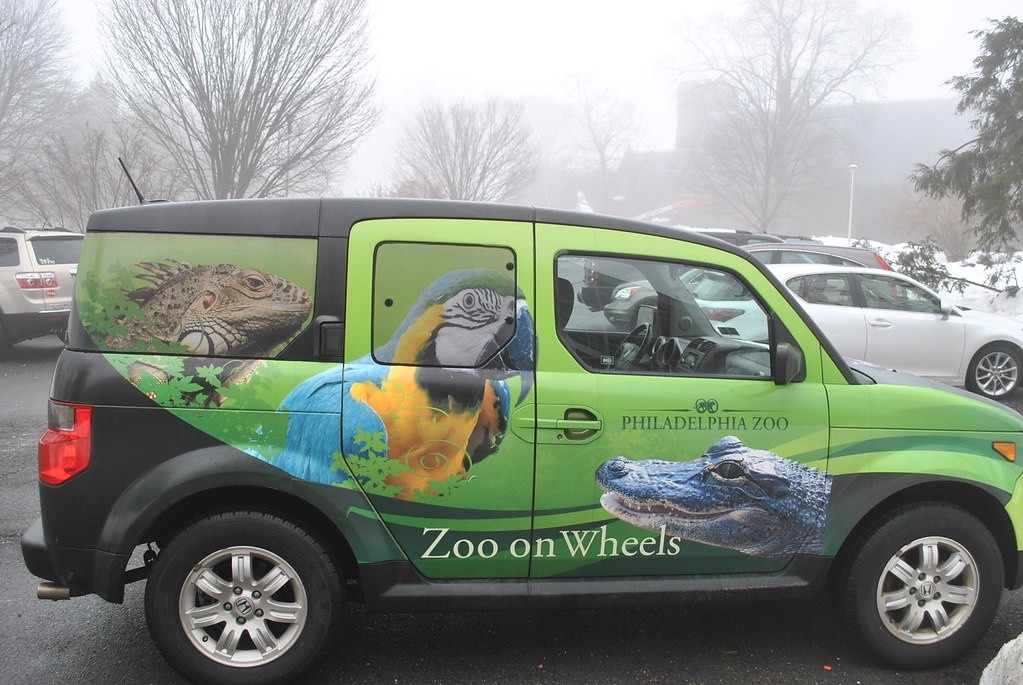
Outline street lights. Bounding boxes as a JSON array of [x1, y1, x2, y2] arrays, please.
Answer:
[[847, 162, 859, 247]]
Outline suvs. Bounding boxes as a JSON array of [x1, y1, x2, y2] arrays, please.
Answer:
[[686, 261, 1023, 401], [575, 227, 828, 312], [0, 224, 85, 365], [11, 194, 1023, 685], [601, 237, 902, 333]]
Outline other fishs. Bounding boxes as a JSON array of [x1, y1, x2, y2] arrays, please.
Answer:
[[595, 436, 833, 558]]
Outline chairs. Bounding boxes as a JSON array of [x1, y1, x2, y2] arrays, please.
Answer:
[[840, 284, 854, 305], [811, 277, 828, 303], [559, 278, 576, 352]]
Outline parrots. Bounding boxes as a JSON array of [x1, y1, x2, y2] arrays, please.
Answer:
[[240, 269, 539, 500]]
